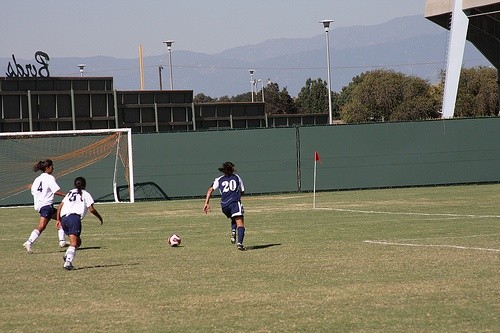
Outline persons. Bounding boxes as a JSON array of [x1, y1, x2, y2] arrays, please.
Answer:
[[23, 159, 71, 253], [55, 177, 103, 270], [203, 162, 247, 251]]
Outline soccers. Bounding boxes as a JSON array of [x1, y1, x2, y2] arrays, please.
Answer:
[[168, 234, 182, 247]]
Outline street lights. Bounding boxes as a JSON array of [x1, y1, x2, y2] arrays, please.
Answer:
[[318, 20, 335, 124], [258, 79, 264, 101], [248, 70, 254, 102], [77, 64, 86, 77], [162, 41, 175, 90]]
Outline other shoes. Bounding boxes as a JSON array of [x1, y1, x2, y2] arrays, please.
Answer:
[[237, 242, 246, 251], [63, 255, 66, 261], [23, 239, 33, 254], [59, 241, 71, 248], [230, 231, 236, 244], [64, 261, 74, 270]]
